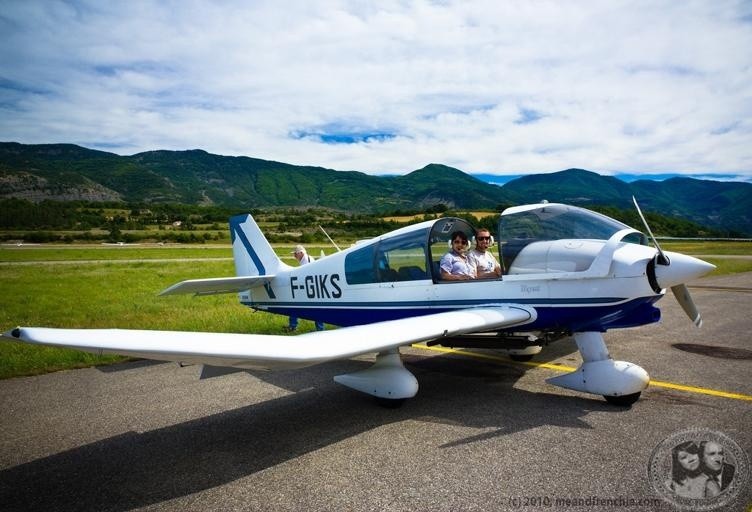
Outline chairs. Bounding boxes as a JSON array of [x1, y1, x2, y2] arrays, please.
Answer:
[[428, 260, 441, 277], [399, 265, 423, 280]]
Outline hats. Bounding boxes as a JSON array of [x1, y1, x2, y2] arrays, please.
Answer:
[[289, 244, 304, 254]]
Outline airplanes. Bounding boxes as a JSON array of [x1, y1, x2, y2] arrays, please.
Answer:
[[0, 195, 716, 410]]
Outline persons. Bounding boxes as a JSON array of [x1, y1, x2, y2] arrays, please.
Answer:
[[670, 440, 721, 498], [470, 228, 504, 280], [697, 439, 733, 492], [286, 244, 325, 332], [438, 230, 474, 280]]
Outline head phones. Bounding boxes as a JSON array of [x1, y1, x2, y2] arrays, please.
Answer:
[[472, 236, 495, 245], [448, 239, 471, 249]]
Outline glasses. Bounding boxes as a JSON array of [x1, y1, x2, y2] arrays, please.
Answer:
[[476, 236, 490, 241], [455, 240, 466, 245]]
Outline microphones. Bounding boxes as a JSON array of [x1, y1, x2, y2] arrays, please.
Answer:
[[485, 245, 494, 249], [458, 248, 467, 251]]
